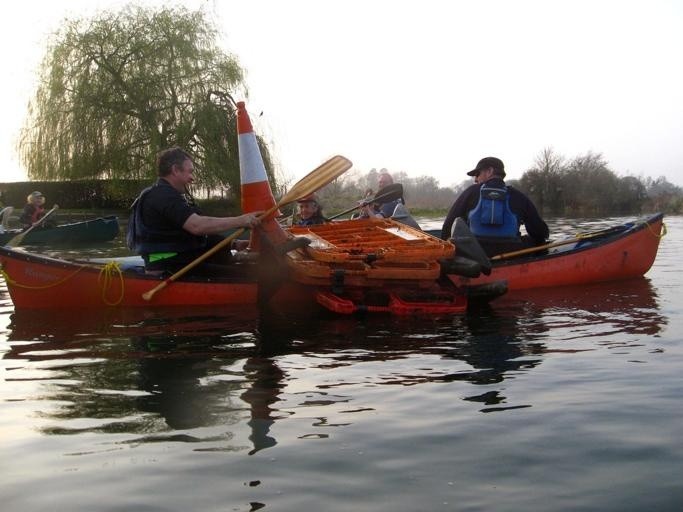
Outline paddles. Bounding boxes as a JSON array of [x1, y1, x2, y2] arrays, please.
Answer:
[[488, 222, 634, 262], [5, 206, 57, 246], [142, 156, 353, 301], [326, 183, 403, 220]]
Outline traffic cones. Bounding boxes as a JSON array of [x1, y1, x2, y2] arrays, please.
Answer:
[[234, 100, 311, 265]]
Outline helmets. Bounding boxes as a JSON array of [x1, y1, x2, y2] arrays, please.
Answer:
[[296, 190, 318, 203]]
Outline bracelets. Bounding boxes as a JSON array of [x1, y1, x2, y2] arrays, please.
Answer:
[[232, 238, 240, 248]]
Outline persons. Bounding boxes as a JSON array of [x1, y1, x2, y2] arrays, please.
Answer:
[[18, 192, 57, 230], [438, 157, 550, 262], [124, 147, 267, 278], [293, 191, 330, 225], [349, 172, 402, 222]]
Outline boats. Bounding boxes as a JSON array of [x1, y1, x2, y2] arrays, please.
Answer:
[[0, 210, 668, 310], [0, 214, 122, 248]]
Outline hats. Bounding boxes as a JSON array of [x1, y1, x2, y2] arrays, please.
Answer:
[[466, 156, 504, 176], [29, 191, 44, 199]]
